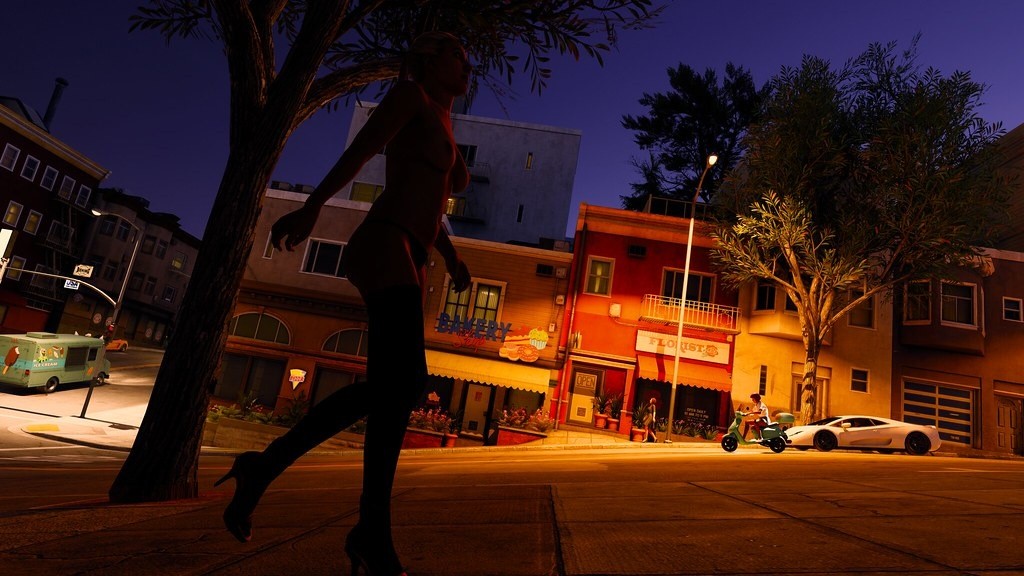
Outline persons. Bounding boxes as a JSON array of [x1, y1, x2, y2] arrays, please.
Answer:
[[743, 394, 769, 440], [212, 31, 472, 576], [643, 397, 658, 443]]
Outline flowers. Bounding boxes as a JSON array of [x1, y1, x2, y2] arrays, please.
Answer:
[[657, 417, 718, 439], [407, 404, 460, 435], [493, 403, 556, 433]]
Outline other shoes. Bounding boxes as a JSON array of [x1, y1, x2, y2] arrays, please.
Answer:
[[654, 438, 658, 443], [643, 438, 648, 443]]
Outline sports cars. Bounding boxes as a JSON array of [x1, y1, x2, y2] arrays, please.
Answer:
[[785, 415, 942, 455]]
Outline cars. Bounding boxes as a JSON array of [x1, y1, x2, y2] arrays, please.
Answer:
[[92, 335, 128, 353]]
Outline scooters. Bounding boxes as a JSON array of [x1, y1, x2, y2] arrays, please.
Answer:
[[720, 404, 796, 453]]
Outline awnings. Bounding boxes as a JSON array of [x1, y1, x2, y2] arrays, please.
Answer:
[[634, 355, 732, 392], [425, 348, 550, 395]]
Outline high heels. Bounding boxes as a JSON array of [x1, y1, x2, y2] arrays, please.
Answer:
[[345, 524, 411, 576], [214, 451, 265, 543]]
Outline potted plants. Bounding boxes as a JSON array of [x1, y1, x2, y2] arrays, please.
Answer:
[[202, 389, 369, 452], [590, 391, 645, 443]]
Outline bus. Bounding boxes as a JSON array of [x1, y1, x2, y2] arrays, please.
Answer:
[[0, 332, 112, 393]]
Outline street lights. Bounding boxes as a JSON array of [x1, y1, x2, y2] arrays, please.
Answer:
[[79, 209, 144, 420], [661, 153, 719, 442]]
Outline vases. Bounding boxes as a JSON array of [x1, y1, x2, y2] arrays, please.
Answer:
[[496, 425, 548, 446], [401, 426, 459, 450]]
